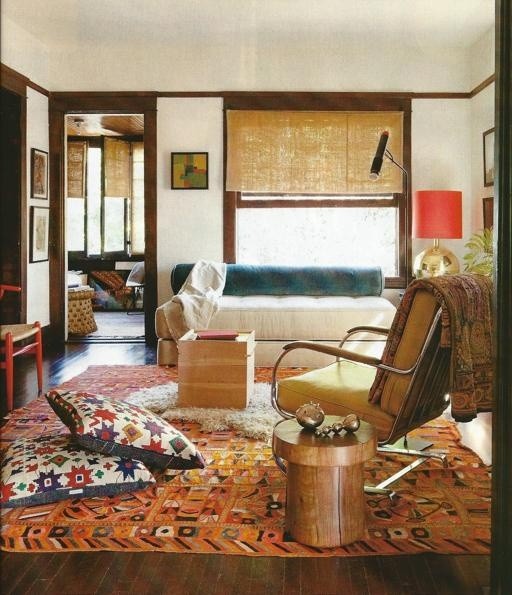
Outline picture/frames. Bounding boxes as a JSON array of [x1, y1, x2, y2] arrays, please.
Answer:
[[172, 152, 208, 189], [483, 198, 494, 256], [30, 207, 49, 263], [483, 127, 495, 187], [31, 149, 48, 200]]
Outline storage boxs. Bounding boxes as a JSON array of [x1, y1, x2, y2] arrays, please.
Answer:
[[176, 329, 257, 409]]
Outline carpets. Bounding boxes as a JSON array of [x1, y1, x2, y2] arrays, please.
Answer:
[[123, 382, 288, 443], [0, 364, 492, 559]]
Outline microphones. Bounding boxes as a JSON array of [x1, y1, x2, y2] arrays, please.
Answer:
[[370, 132, 390, 181]]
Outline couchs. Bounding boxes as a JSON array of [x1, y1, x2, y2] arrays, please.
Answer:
[[154, 264, 397, 374]]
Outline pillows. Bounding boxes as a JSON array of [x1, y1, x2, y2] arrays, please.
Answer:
[[0, 437, 157, 508], [45, 388, 207, 470]]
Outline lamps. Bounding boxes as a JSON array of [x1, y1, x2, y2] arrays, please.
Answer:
[[412, 190, 462, 280], [369, 131, 409, 299]]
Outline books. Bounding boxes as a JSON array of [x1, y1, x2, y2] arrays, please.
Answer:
[[197, 330, 239, 339]]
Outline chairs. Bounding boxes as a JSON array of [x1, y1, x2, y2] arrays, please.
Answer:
[[0, 285, 42, 410], [271, 271, 492, 506]]
